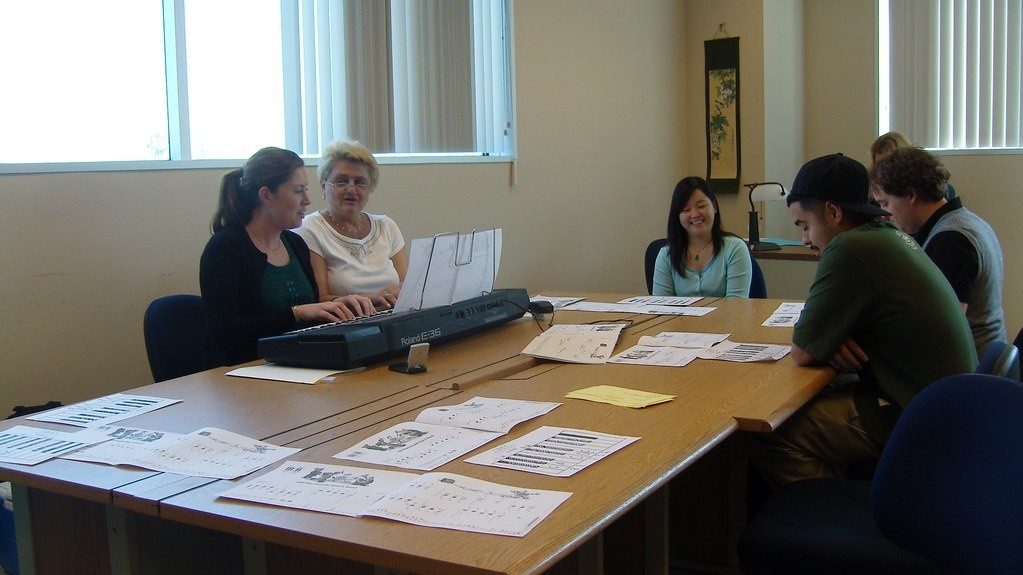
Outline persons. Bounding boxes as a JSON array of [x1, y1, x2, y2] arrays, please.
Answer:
[[869, 146, 1008, 360], [871, 131, 955, 201], [291, 141, 408, 309], [748, 153, 978, 482], [199, 147, 377, 363], [652, 177, 752, 299]]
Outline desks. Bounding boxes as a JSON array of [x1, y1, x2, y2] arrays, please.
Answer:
[[752, 245, 819, 261], [0, 290, 834, 575]]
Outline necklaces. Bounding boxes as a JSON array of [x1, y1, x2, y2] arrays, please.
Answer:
[[327, 209, 363, 232], [692, 239, 712, 260]]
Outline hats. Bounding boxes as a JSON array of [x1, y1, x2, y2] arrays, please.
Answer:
[[791, 153, 893, 216]]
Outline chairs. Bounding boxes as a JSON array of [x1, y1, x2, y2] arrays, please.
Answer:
[[645, 238, 767, 298], [144, 294, 202, 382], [747, 343, 1019, 550], [872, 377, 1023, 575]]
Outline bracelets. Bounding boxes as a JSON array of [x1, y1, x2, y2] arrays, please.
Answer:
[[331, 297, 339, 302]]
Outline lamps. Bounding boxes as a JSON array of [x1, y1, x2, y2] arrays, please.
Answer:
[[743, 183, 792, 249]]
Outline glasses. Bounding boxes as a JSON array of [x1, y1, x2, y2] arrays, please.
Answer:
[[326, 181, 372, 194]]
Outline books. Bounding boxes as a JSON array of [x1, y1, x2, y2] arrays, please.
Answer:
[[520, 324, 626, 365]]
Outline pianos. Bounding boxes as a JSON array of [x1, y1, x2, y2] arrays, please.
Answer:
[[256, 288, 531, 370]]
